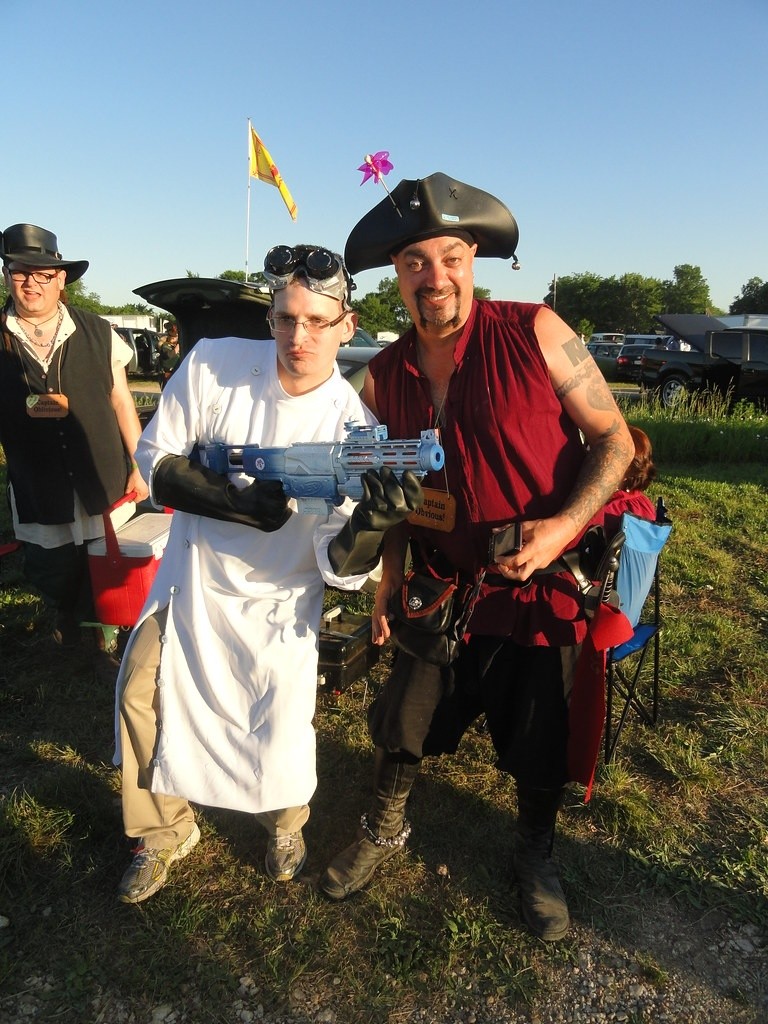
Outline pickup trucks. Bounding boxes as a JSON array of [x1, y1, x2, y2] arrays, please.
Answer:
[[639, 314, 767, 410]]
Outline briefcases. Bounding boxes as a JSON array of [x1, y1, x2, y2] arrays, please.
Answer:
[[87, 492, 174, 627], [317, 605, 380, 695]]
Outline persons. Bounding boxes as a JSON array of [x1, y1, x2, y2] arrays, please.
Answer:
[[638, 337, 691, 394], [578, 333, 587, 348], [610, 335, 617, 343], [135, 335, 148, 380], [156, 322, 180, 389], [0, 224, 149, 669], [113, 246, 425, 902], [585, 425, 659, 652], [109, 323, 129, 375], [318, 171, 635, 941]]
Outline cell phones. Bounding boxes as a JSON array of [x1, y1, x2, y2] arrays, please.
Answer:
[[487, 522, 523, 564]]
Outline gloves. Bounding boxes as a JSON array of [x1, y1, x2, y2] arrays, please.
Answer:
[[153, 454, 294, 533], [328, 467, 425, 577]]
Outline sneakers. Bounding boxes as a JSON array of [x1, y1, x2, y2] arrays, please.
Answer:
[[511, 847, 570, 941], [117, 822, 201, 903], [265, 829, 307, 882], [319, 836, 405, 900]]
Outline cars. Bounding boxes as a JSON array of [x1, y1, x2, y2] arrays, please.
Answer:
[[113, 327, 164, 375], [615, 344, 658, 382], [131, 278, 387, 377], [585, 341, 624, 381]]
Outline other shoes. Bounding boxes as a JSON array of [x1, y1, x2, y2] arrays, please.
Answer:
[[117, 629, 134, 659], [57, 615, 103, 674]]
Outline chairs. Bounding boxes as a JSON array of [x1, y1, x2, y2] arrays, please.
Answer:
[[603, 495, 673, 766]]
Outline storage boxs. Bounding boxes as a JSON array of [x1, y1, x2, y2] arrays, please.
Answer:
[[88, 513, 173, 627]]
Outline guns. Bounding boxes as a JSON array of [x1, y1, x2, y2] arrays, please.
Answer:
[[188, 412, 447, 519]]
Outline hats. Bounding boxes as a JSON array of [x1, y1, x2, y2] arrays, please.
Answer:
[[0, 224, 89, 285], [344, 171, 522, 290]]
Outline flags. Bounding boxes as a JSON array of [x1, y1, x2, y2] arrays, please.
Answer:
[[248, 124, 297, 221]]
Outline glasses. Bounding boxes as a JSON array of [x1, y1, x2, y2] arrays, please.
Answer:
[[8, 269, 62, 284], [266, 304, 348, 334], [263, 245, 341, 288]]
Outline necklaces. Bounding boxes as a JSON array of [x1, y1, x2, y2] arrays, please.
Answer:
[[15, 301, 63, 347]]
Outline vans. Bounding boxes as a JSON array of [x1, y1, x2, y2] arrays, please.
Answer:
[[589, 333, 625, 344]]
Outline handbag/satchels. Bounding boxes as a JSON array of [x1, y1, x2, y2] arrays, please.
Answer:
[[386, 571, 458, 639]]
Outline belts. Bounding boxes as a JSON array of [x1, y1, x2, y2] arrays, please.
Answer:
[[429, 547, 583, 584]]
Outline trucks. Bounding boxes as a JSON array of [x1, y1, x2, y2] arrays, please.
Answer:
[[377, 332, 399, 347], [98, 314, 169, 333]]
